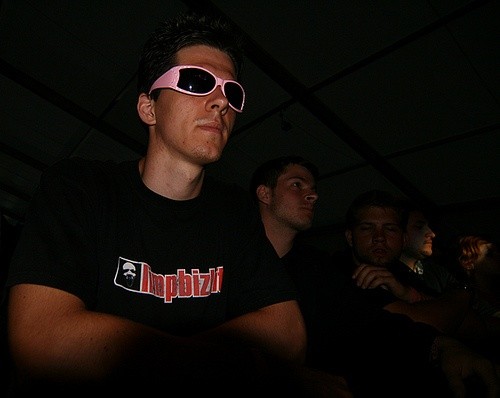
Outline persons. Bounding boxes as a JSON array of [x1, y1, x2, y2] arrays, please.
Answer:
[[383, 199, 471, 305], [450, 230, 500, 332], [218, 154, 499, 398], [2, 16, 308, 397], [337, 187, 473, 333]]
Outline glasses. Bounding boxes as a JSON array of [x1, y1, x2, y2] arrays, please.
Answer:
[[149, 64, 246, 113]]
